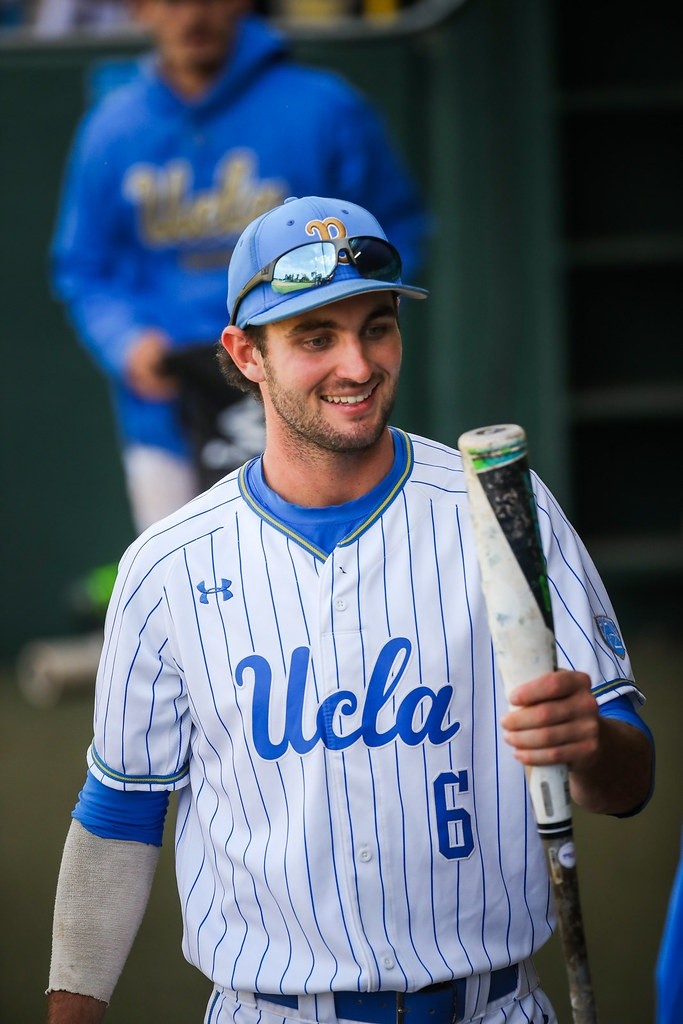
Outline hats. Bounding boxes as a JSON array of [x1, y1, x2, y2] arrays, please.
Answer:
[[227, 194, 427, 329]]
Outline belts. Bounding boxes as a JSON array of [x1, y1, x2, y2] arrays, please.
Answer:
[[252, 961, 519, 1024]]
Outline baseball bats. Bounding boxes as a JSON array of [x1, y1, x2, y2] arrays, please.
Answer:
[[457, 424, 598, 1024]]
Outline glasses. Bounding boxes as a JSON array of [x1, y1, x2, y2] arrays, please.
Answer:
[[230, 236, 404, 328]]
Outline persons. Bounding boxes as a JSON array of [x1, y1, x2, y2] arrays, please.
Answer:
[[49, 0, 427, 540], [46, 195, 656, 1024]]
